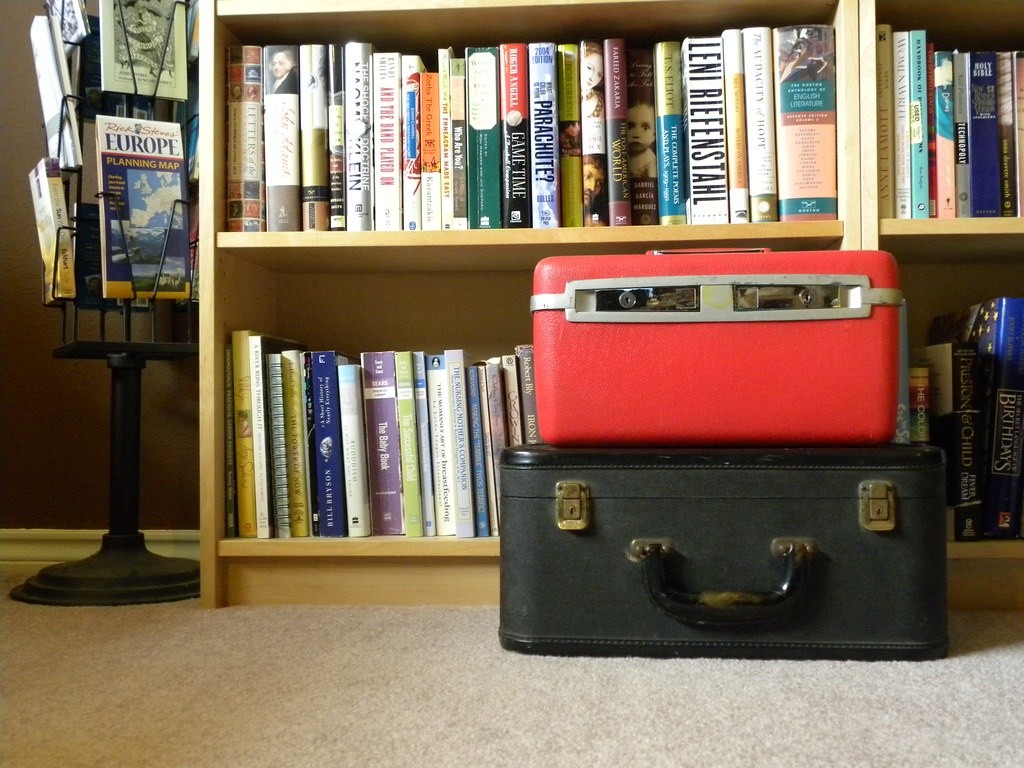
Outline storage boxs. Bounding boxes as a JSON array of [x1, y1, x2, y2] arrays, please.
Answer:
[[530, 247, 902, 447]]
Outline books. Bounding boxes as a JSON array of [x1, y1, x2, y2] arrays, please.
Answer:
[[28, 0, 91, 308], [97, 0, 189, 103], [94, 114, 192, 299], [227, 24, 839, 234], [222, 326, 542, 537], [876, 22, 1024, 219], [909, 342, 1023, 542]]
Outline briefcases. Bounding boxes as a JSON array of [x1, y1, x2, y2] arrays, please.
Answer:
[[499, 446, 951, 662], [530, 242, 906, 446]]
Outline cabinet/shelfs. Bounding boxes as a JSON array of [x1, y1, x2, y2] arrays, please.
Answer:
[[9, 0, 199, 606], [199, 0, 1024, 609]]
